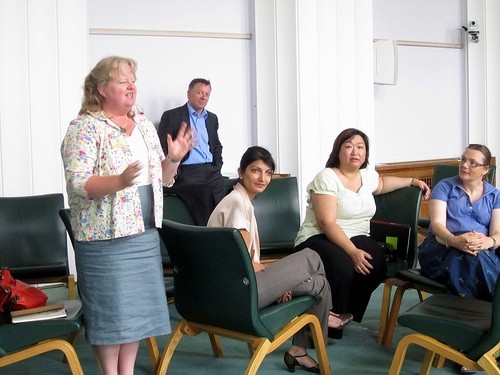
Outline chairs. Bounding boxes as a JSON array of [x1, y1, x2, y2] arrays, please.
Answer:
[[0, 164, 500, 375]]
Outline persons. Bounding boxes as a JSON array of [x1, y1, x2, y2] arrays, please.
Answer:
[[293, 129, 432, 340], [60, 56, 201, 375], [158, 79, 226, 226], [417, 144, 500, 375], [205, 146, 354, 372]]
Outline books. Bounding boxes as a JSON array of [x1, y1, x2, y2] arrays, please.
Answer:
[[10, 304, 67, 323]]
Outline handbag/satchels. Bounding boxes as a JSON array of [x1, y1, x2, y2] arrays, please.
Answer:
[[0, 267, 48, 313]]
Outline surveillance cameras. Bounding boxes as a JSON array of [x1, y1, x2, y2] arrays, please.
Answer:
[[468, 20, 479, 31]]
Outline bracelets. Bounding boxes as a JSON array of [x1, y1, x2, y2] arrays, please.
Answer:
[[168, 157, 180, 163], [491, 237, 496, 248], [410, 178, 414, 187]]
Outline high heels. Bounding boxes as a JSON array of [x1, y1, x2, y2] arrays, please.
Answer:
[[284, 351, 331, 372], [328, 311, 354, 330]]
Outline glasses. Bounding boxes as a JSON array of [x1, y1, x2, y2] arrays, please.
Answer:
[[458, 157, 487, 167]]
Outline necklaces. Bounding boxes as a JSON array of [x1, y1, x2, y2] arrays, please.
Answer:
[[104, 111, 127, 133]]
